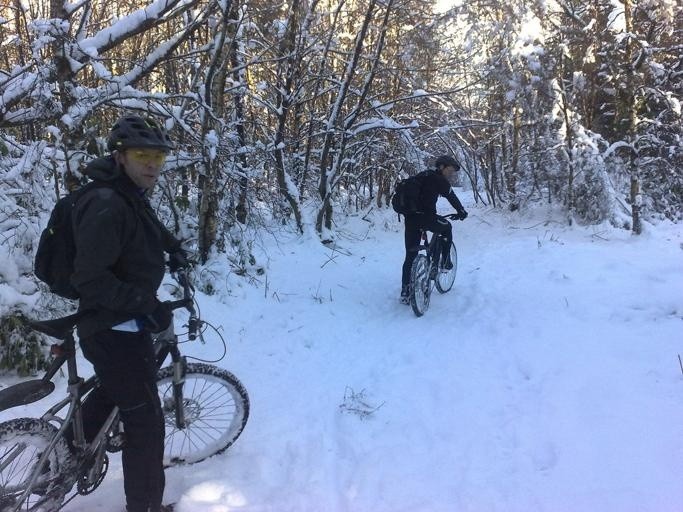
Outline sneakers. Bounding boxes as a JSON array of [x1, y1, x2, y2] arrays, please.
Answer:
[[22, 462, 50, 497], [399, 292, 411, 305], [441, 262, 452, 272]]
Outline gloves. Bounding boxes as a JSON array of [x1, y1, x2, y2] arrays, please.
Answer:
[[168, 247, 188, 270], [135, 299, 173, 336], [456, 207, 468, 220]]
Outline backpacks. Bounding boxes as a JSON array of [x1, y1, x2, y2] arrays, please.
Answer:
[[391, 169, 437, 221], [34, 182, 118, 302]]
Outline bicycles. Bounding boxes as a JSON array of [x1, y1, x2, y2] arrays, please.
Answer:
[[0, 259, 250, 512], [407, 214, 458, 317]]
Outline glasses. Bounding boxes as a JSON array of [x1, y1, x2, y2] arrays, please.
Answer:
[[123, 151, 167, 166]]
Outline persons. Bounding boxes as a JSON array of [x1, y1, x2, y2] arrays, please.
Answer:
[[399, 155, 468, 306], [67, 115, 195, 512]]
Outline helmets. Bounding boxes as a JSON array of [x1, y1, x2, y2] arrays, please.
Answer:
[[107, 115, 177, 151], [434, 156, 460, 172]]
